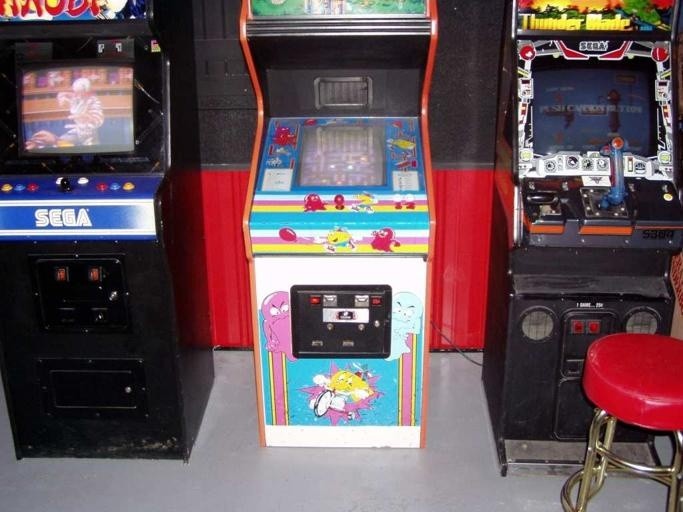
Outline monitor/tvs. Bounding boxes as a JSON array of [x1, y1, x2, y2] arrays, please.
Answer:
[[530, 53, 659, 159], [289, 117, 390, 191], [16, 61, 139, 157]]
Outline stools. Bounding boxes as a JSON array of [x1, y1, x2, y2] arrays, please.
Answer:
[[560, 332, 683, 508]]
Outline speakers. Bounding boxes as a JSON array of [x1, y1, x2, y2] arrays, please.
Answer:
[[501, 274, 675, 442]]
